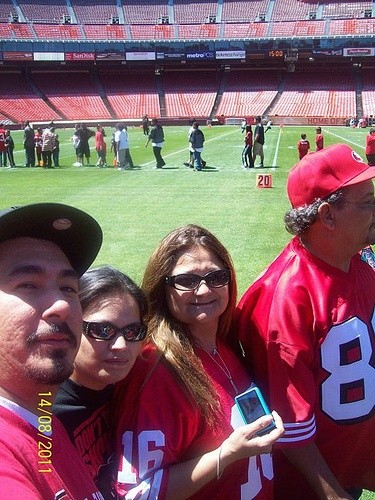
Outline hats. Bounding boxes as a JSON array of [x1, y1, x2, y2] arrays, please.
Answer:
[[0, 202, 104, 278], [287, 144, 375, 209]]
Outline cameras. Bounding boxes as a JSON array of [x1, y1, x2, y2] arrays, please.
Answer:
[[235, 387, 275, 438]]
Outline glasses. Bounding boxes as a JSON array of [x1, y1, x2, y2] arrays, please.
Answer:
[[164, 270, 231, 291], [81, 320, 148, 342], [340, 200, 375, 209]]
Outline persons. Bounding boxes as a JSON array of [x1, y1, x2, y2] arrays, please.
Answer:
[[0, 203, 105, 500], [71, 123, 96, 166], [145, 118, 165, 169], [350, 116, 367, 129], [53, 266, 148, 500], [109, 225, 285, 500], [4, 130, 15, 168], [236, 144, 375, 500], [264, 119, 273, 133], [183, 119, 206, 172], [365, 128, 375, 167], [23, 120, 60, 168], [315, 126, 323, 152], [110, 123, 133, 168], [142, 115, 150, 136], [298, 133, 310, 160], [242, 116, 265, 168], [0, 123, 8, 167], [95, 124, 107, 168]]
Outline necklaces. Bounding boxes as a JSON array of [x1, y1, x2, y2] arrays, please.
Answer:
[[194, 341, 237, 394]]
